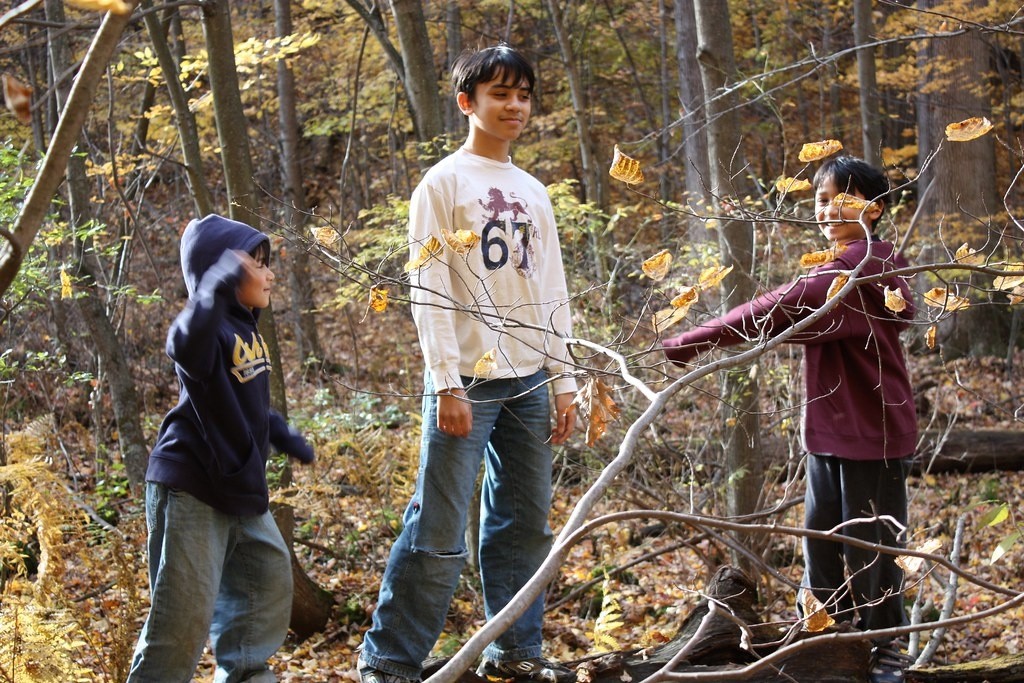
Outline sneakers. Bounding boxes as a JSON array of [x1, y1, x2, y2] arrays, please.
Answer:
[[868, 642, 906, 682], [357, 649, 423, 683], [475, 657, 576, 683]]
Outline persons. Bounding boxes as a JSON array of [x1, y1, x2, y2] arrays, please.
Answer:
[[662, 154, 918, 683], [357, 46, 579, 683], [125, 214, 315, 683]]
